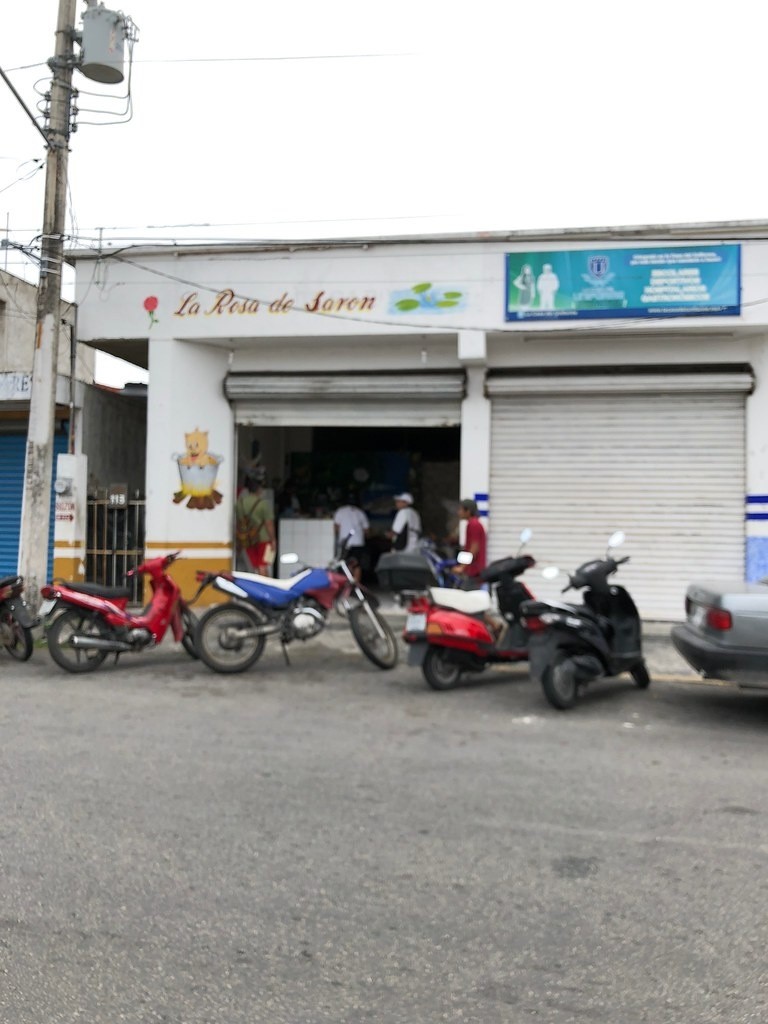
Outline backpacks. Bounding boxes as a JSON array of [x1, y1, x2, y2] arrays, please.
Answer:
[[236, 498, 263, 548]]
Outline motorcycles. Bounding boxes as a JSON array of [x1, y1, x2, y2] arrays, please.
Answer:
[[0, 575, 41, 662], [520, 531, 651, 709], [40, 550, 199, 674], [396, 528, 543, 691], [194, 528, 398, 676]]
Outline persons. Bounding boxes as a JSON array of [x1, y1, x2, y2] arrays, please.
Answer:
[[390, 493, 422, 552], [236, 464, 276, 578], [537, 263, 559, 308], [333, 491, 372, 580], [452, 500, 486, 590], [513, 264, 536, 307]]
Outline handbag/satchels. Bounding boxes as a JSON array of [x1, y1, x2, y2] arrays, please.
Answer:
[[391, 524, 408, 550], [263, 544, 275, 565]]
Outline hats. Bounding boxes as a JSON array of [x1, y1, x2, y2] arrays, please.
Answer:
[[394, 492, 414, 504], [462, 499, 479, 515]]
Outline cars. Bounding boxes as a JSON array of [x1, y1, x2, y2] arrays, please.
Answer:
[[669, 577, 768, 691]]
